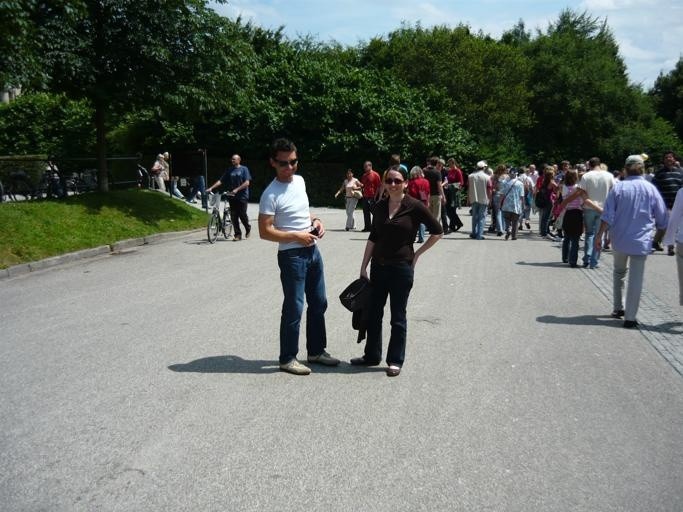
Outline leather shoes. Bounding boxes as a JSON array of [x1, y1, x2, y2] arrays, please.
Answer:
[[388, 368, 400, 376], [350, 358, 380, 366], [525, 223, 530, 229], [498, 232, 502, 236]]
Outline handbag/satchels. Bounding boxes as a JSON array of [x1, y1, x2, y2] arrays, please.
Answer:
[[494, 192, 505, 210], [351, 189, 363, 199]]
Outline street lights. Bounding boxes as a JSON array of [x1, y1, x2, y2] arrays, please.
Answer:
[[197, 149, 208, 188]]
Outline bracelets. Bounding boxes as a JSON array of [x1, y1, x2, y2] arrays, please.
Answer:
[[310, 218, 323, 226]]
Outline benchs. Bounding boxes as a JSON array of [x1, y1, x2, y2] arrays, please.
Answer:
[[0, 154, 151, 203]]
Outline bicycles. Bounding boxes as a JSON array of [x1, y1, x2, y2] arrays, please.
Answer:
[[202, 191, 235, 245]]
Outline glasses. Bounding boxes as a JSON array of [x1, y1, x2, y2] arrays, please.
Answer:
[[274, 159, 298, 166], [385, 178, 405, 184]]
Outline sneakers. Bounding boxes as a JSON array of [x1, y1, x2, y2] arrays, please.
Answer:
[[612, 310, 633, 327], [246, 227, 251, 238], [280, 358, 311, 375], [307, 351, 340, 365], [233, 236, 241, 241]]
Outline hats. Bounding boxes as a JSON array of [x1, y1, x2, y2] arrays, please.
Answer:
[[477, 161, 487, 168]]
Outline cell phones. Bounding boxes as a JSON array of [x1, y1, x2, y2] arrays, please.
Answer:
[[309, 229, 318, 236]]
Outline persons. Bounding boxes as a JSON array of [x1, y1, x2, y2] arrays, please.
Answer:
[[349, 165, 445, 377], [536, 157, 626, 250], [592, 154, 669, 330], [258, 137, 341, 375], [204, 153, 252, 242], [664, 183, 682, 309], [643, 154, 681, 256], [359, 161, 384, 232], [408, 157, 463, 243], [561, 157, 616, 268], [376, 154, 409, 204], [335, 168, 362, 231], [467, 161, 539, 240], [151, 149, 208, 210]]
